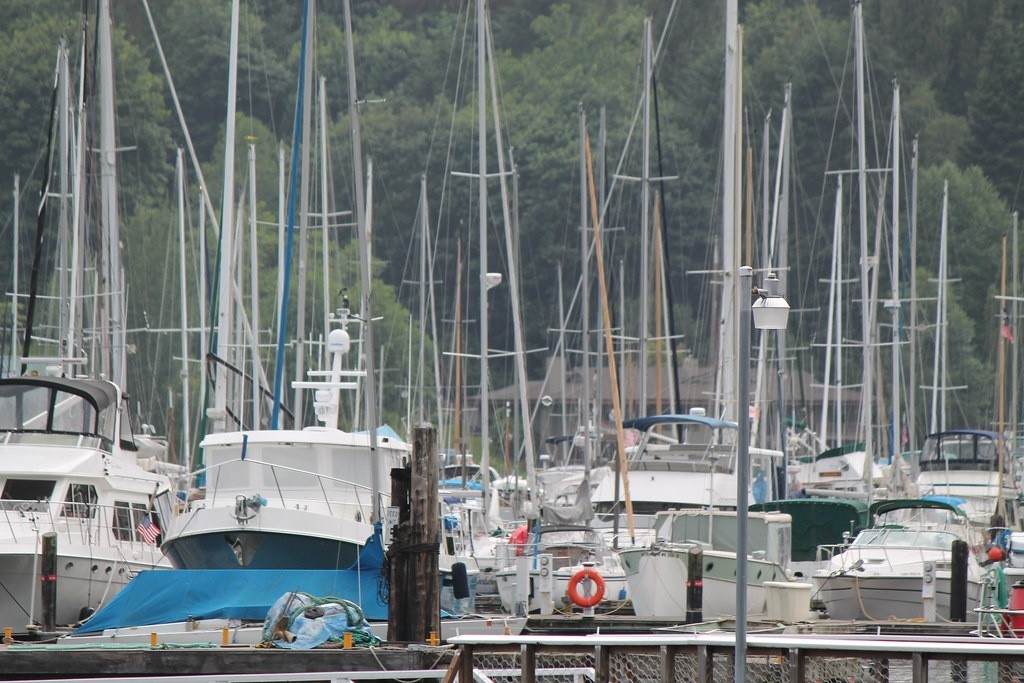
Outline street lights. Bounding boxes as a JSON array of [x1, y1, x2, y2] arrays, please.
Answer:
[[735, 264, 790, 682]]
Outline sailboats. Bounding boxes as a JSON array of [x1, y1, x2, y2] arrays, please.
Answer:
[[0, 1, 1024, 646]]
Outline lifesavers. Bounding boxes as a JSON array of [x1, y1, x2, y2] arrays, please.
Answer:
[[567, 568, 606, 608]]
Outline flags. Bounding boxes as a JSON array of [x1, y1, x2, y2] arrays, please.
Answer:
[[1002, 309, 1014, 343], [138, 518, 160, 544]]
